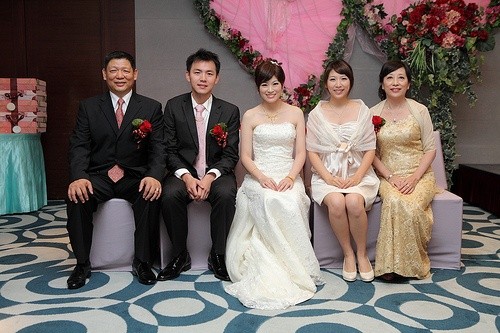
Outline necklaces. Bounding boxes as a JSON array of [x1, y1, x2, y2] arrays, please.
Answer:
[[263, 102, 284, 123], [329, 104, 348, 119], [386, 105, 403, 122]]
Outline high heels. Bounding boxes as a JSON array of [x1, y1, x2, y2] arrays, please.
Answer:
[[342, 258, 357, 282], [358, 255, 375, 283]]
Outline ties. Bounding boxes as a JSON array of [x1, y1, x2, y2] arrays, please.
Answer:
[[192, 104, 206, 180], [107, 98, 125, 183]]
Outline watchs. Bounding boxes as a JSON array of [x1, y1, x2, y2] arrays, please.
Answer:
[[387, 173, 394, 181]]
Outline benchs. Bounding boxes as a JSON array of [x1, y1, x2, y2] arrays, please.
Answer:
[[90, 129, 243, 270], [306, 132, 463, 271]]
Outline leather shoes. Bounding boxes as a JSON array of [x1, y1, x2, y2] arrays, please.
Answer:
[[66, 263, 92, 289], [157, 249, 192, 282], [207, 252, 231, 281], [131, 259, 157, 285]]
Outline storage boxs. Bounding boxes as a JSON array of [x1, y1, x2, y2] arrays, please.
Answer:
[[0, 78, 47, 133]]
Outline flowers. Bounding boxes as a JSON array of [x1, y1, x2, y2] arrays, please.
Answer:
[[192, 0, 500, 191], [132, 119, 150, 144]]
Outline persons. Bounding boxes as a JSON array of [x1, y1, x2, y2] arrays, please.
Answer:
[[155, 49, 234, 283], [236, 58, 322, 298], [305, 58, 378, 284], [63, 50, 166, 288], [368, 59, 438, 284]]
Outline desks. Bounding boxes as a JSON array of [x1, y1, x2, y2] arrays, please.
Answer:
[[0, 133, 48, 216]]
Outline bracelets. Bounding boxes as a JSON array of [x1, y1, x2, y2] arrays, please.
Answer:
[[285, 176, 295, 183]]
